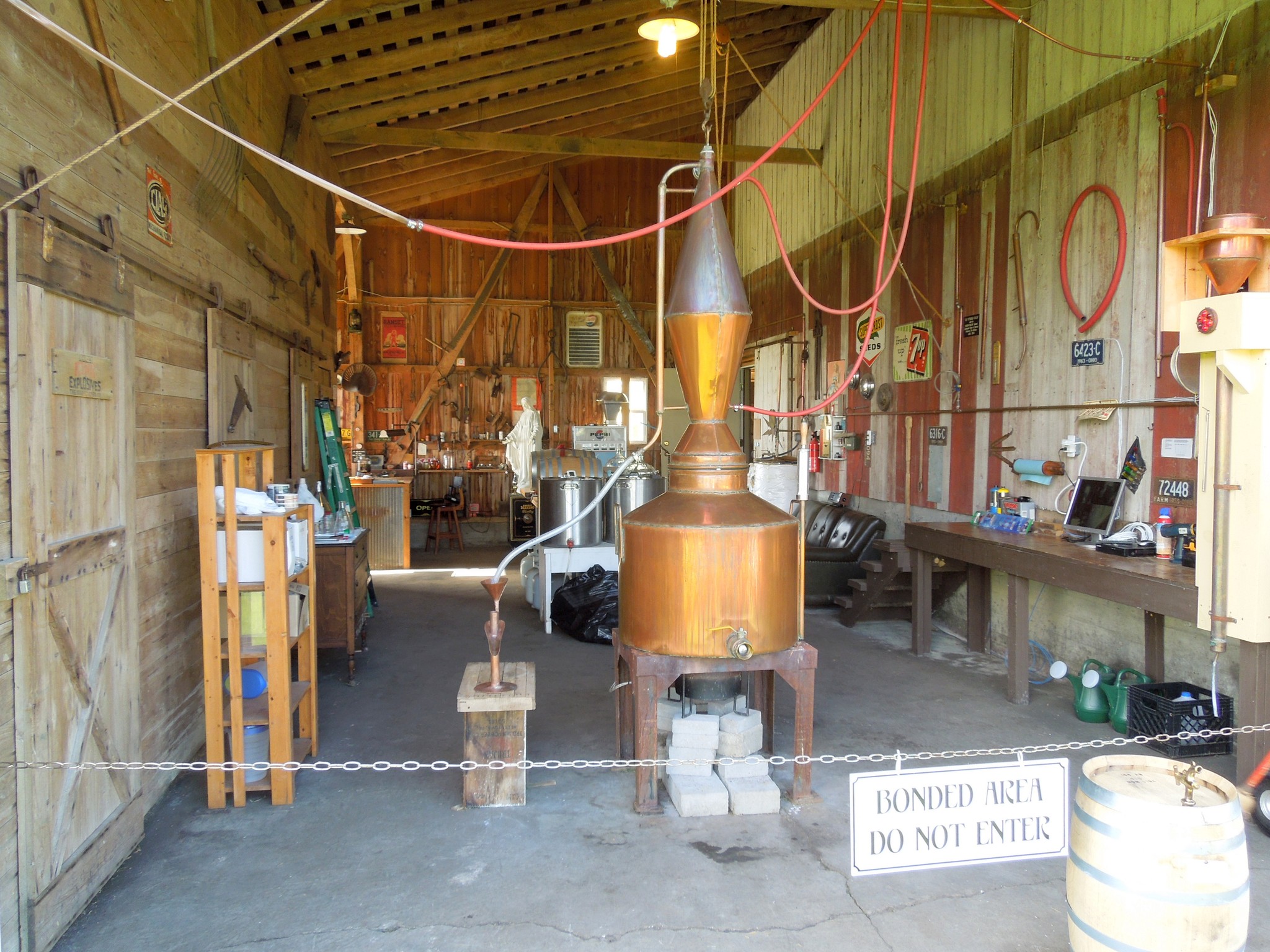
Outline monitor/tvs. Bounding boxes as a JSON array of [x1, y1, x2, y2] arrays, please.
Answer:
[[1063, 475, 1126, 535]]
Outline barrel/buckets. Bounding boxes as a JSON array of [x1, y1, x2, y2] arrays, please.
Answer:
[[1065, 753, 1250, 952], [539, 471, 667, 550]]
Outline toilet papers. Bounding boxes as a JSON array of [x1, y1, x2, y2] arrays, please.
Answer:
[[1012, 455, 1055, 487]]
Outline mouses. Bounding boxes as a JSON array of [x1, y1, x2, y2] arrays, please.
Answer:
[[1138, 540, 1156, 547]]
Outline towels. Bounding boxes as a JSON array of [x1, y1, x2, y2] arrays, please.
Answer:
[[215, 484, 285, 519]]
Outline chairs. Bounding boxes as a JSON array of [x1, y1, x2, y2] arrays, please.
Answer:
[[427, 476, 466, 555]]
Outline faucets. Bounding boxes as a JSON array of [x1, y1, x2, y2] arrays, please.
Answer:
[[1171, 758, 1207, 798]]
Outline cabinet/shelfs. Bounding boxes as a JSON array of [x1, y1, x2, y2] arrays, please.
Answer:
[[417, 438, 514, 474], [193, 444, 321, 806], [316, 525, 370, 677]]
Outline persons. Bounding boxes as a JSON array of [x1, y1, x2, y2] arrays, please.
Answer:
[[506, 395, 543, 493]]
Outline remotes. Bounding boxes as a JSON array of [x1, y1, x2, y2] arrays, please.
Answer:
[[1096, 539, 1132, 545]]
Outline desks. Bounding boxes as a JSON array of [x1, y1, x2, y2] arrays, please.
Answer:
[[904, 522, 1268, 783], [537, 543, 621, 635], [349, 470, 415, 569]]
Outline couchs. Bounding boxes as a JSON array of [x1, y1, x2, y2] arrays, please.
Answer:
[[790, 498, 886, 605]]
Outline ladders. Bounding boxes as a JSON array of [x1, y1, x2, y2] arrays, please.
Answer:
[[314, 396, 378, 616]]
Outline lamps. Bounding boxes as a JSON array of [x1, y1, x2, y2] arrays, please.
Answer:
[[638, 1, 699, 59], [334, 215, 367, 235]]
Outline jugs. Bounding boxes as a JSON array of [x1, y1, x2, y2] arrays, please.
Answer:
[[1082, 667, 1155, 734], [1049, 659, 1115, 724]]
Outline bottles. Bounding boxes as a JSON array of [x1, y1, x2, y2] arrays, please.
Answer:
[[299, 478, 354, 539], [1155, 508, 1174, 559], [442, 447, 456, 469]]
